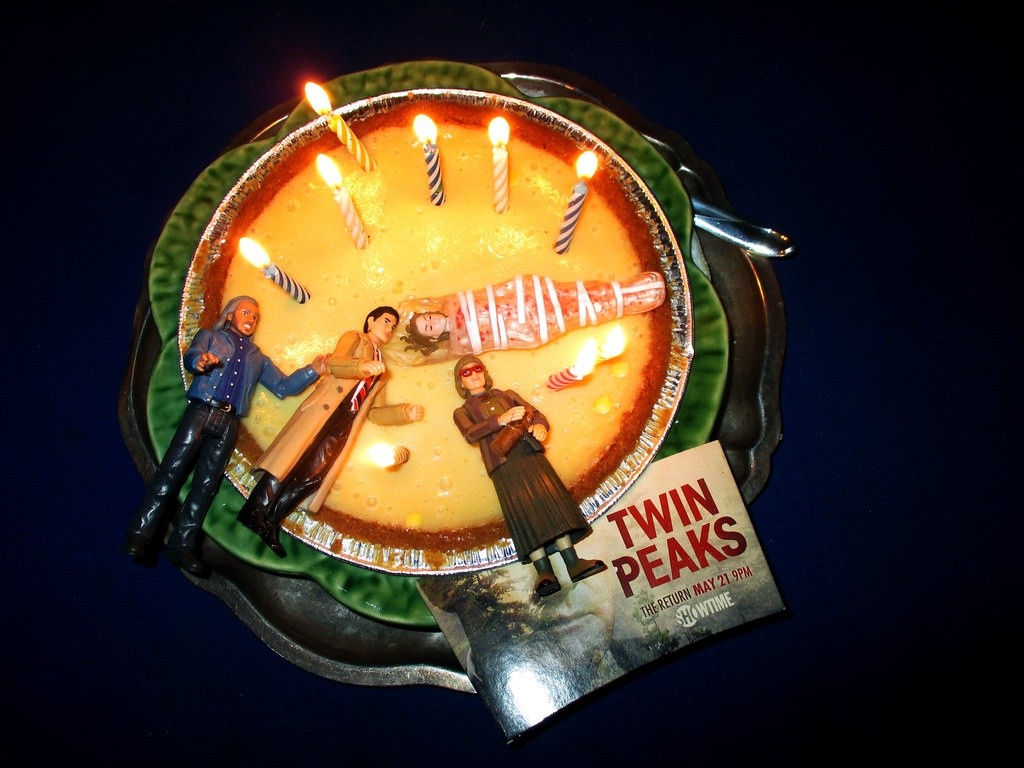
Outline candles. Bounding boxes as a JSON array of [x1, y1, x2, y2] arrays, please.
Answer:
[[548, 327, 628, 392], [487, 118, 512, 214], [370, 443, 409, 468], [239, 234, 309, 304], [553, 150, 597, 255], [305, 81, 378, 171], [413, 112, 446, 208], [314, 153, 369, 250]]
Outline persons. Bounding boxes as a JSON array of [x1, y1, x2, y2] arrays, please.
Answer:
[[236, 306, 423, 558], [453, 357, 607, 597], [399, 272, 666, 356], [125, 296, 330, 579]]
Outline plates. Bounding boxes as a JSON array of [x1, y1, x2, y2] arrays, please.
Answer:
[[145, 61, 730, 627]]
